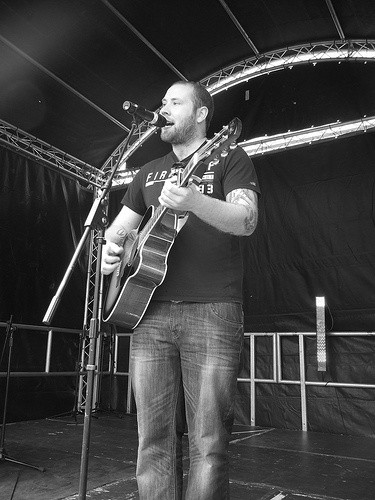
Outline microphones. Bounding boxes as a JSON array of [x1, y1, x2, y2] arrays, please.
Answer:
[[123, 101, 166, 127]]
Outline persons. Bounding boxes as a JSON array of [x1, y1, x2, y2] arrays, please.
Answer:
[[101, 81, 261, 500]]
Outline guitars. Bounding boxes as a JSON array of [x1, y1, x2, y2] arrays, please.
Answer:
[[102, 116, 242, 331]]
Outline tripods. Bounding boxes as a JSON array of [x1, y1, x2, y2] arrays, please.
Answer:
[[0, 315, 46, 472]]
